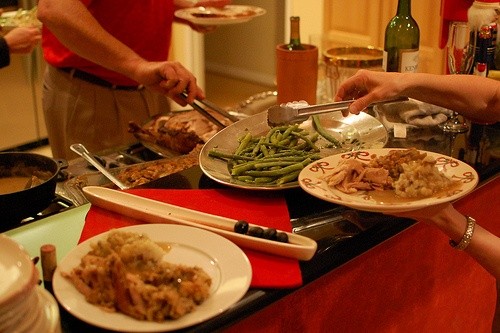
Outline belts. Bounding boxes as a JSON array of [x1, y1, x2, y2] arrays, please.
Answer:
[[61, 67, 145, 90]]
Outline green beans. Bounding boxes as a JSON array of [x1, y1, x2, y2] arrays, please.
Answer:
[[208, 114, 342, 187]]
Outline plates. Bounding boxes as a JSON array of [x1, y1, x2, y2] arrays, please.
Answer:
[[0, 233, 62, 333], [51, 223, 252, 333], [199, 104, 389, 190], [175, 5, 266, 25], [0, 11, 41, 32], [298, 148, 478, 213]]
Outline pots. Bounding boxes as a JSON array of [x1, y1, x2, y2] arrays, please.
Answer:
[[0, 151, 69, 222]]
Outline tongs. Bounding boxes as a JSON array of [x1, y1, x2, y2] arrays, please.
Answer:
[[176, 83, 242, 129], [266, 97, 408, 127]]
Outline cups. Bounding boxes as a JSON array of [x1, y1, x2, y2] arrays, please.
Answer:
[[276, 45, 320, 106], [323, 45, 385, 99]]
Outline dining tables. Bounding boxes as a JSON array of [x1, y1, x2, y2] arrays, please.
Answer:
[[0, 73, 500, 333]]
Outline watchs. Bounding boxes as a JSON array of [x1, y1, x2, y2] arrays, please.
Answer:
[[449, 216, 476, 251]]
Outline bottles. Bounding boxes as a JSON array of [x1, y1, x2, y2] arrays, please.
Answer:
[[285, 17, 305, 51], [385, 0, 420, 75]]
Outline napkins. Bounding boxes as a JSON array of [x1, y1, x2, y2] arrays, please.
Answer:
[[78, 188, 303, 287]]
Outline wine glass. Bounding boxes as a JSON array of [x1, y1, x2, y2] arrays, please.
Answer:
[[438, 21, 476, 133]]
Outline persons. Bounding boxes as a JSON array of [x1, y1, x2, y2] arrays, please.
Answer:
[[0, 6, 41, 69], [36, 0, 232, 161], [335, 70, 500, 333]]
